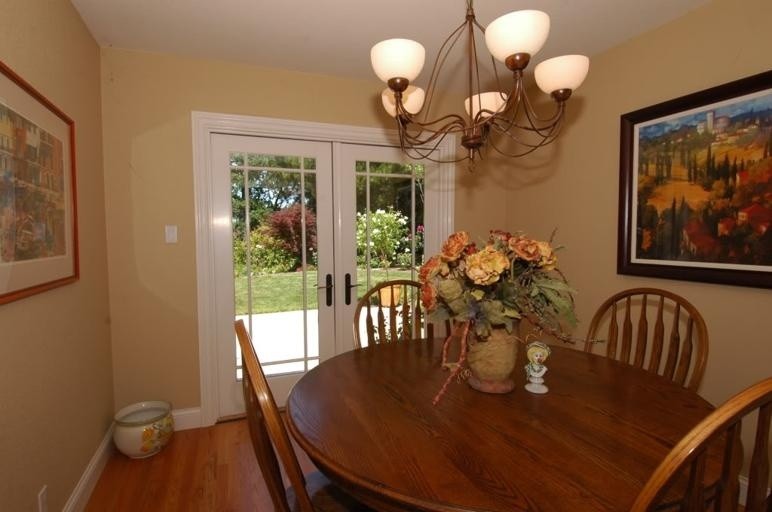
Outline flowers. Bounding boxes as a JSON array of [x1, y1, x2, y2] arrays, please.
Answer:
[[356, 205, 411, 281], [418, 227, 605, 405]]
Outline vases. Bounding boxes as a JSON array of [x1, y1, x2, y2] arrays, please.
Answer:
[[466, 323, 520, 394], [376, 283, 401, 307]]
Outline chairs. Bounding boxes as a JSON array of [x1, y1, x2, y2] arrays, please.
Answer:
[[233, 320, 365, 511], [352, 279, 459, 350], [629, 377, 772, 512], [582, 287, 709, 391]]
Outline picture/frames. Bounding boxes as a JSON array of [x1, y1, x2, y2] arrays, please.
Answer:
[[618, 70, 772, 290], [1, 60, 79, 305]]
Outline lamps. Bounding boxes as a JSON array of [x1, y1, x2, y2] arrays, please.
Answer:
[[369, 0, 591, 166]]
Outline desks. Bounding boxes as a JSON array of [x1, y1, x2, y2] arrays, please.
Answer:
[[287, 335, 743, 511]]
